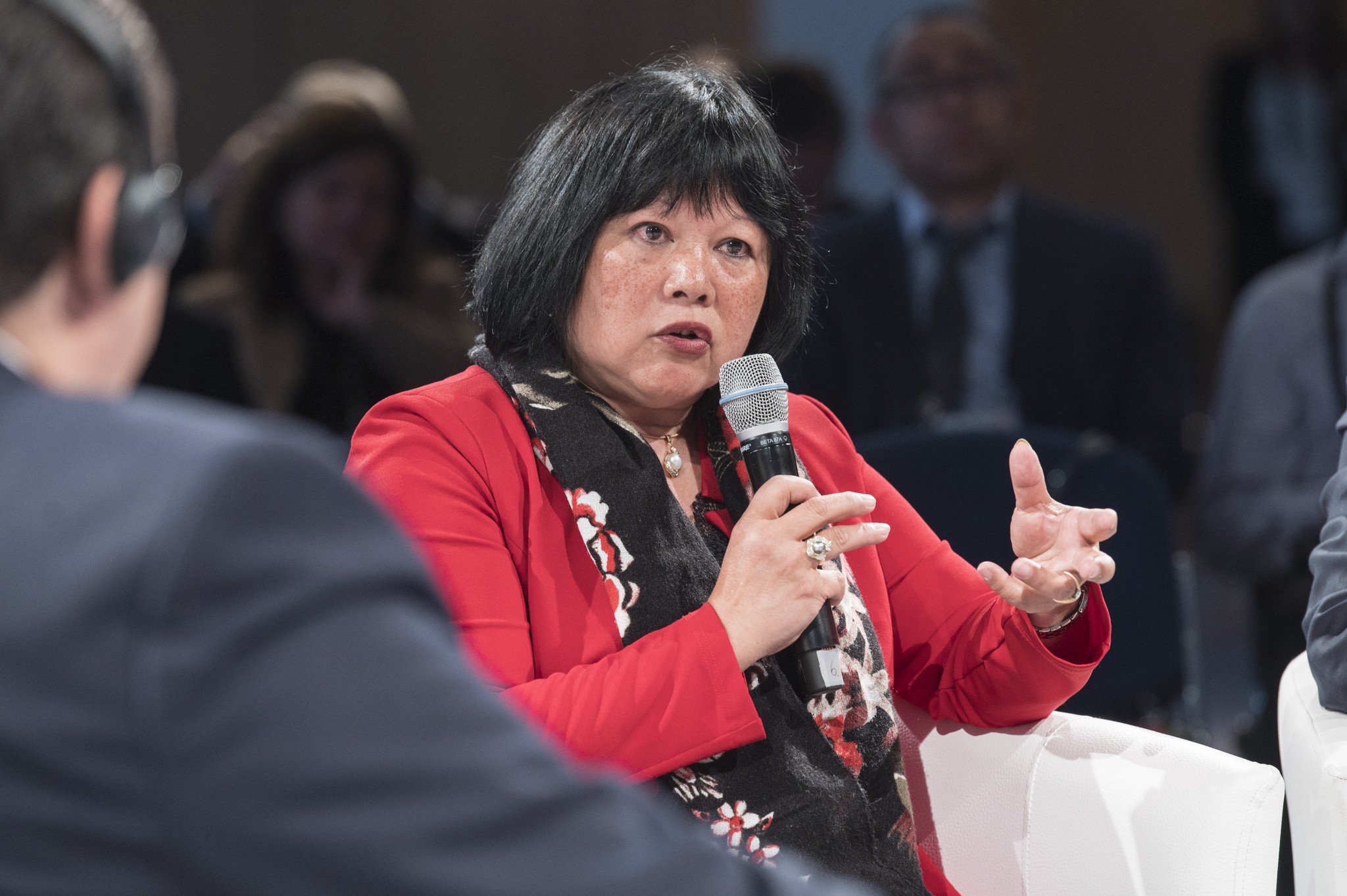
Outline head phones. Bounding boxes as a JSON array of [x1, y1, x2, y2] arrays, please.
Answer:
[[33, 1, 175, 282]]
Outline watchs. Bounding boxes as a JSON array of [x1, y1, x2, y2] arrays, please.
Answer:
[[1031, 585, 1091, 639]]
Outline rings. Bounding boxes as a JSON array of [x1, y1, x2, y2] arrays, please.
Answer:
[[806, 532, 833, 566], [1052, 570, 1082, 605]]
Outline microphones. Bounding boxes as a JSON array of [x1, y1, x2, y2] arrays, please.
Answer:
[[721, 354, 845, 696]]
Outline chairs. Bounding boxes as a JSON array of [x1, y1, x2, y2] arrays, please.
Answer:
[[919, 643, 1347, 896]]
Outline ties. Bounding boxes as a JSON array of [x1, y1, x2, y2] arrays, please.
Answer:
[[919, 220, 997, 411]]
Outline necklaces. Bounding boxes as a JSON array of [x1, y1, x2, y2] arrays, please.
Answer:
[[639, 431, 688, 478]]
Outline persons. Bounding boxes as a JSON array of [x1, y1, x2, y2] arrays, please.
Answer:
[[133, 54, 489, 448], [1200, 234, 1347, 896], [732, 16, 1185, 723], [342, 66, 1121, 896], [1301, 408, 1347, 717], [1, 0, 779, 896]]
